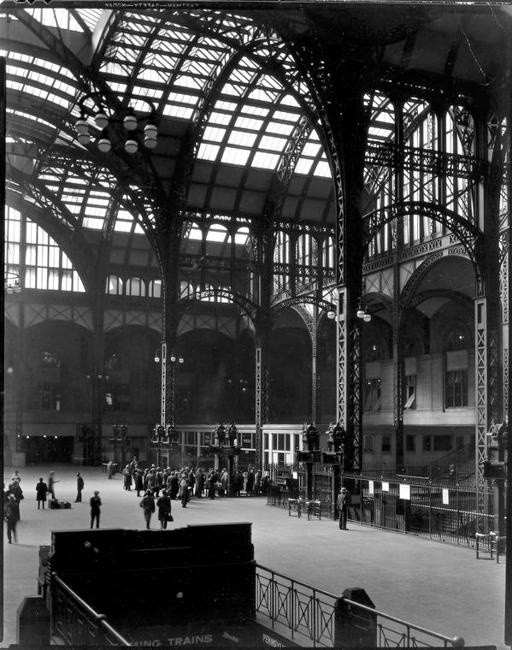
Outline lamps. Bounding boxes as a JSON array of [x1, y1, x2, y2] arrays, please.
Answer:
[[75, 91, 160, 154]]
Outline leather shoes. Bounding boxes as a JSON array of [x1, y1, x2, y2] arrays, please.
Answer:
[[340, 528, 349, 530]]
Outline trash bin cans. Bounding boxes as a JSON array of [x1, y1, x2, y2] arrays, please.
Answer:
[[17, 595, 50, 650], [336, 587, 378, 649]]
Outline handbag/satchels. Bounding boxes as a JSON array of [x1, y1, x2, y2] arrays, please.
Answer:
[[168, 515, 173, 521]]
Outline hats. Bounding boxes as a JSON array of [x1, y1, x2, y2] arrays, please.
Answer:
[[340, 488, 348, 492], [7, 494, 16, 501]]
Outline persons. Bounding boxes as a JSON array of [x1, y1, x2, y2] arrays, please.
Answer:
[[179, 479, 191, 508], [7, 480, 26, 501], [73, 471, 86, 504], [32, 475, 49, 511], [157, 489, 172, 531], [141, 486, 155, 529], [334, 487, 351, 531], [46, 469, 62, 501], [4, 494, 20, 542], [106, 457, 115, 479], [119, 457, 271, 499], [11, 469, 23, 486], [88, 491, 103, 529]]
